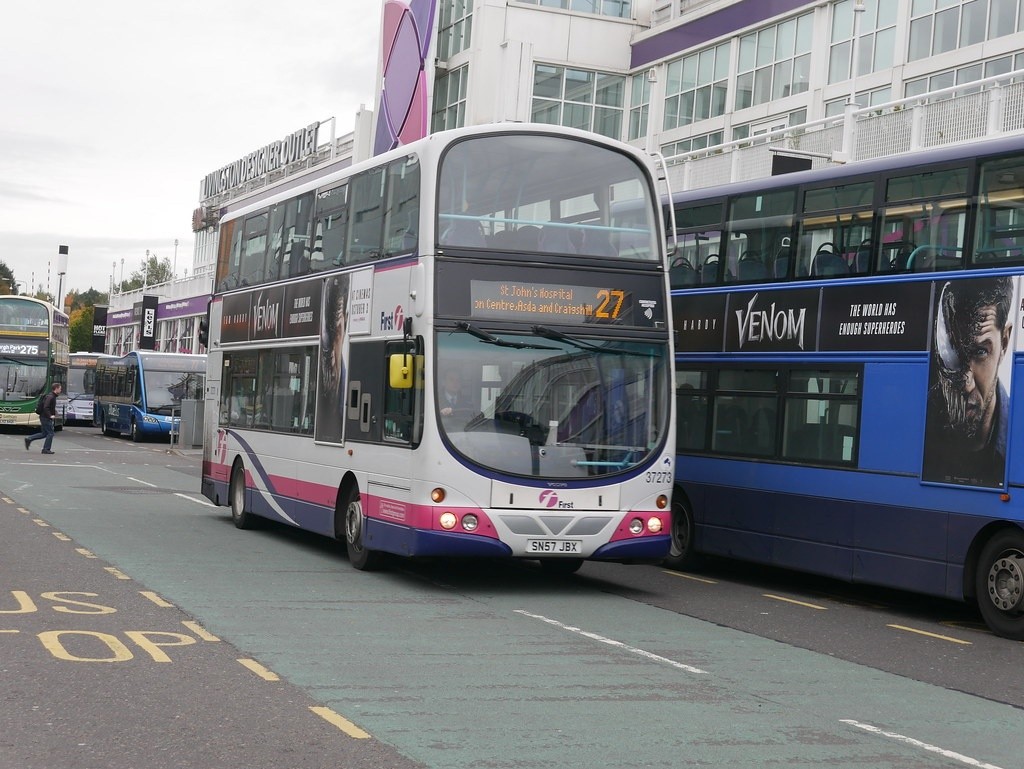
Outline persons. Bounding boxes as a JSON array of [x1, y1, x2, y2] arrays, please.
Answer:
[[438, 367, 467, 417], [321, 274, 350, 412], [25, 383, 61, 454], [928, 276, 1013, 460]]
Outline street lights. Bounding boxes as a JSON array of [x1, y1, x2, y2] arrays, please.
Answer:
[[2, 278, 28, 297], [58, 272, 65, 309]]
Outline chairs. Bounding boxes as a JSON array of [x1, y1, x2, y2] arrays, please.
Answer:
[[669, 239, 917, 287], [443, 217, 619, 258]]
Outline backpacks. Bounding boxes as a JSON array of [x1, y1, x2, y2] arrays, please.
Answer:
[[35, 394, 47, 414]]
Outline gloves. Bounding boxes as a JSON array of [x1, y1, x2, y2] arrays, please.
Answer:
[[441, 407, 454, 417]]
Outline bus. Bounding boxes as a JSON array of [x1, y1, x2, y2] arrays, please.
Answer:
[[611, 130, 1024, 641], [0, 294, 70, 433], [200, 120, 677, 578], [66, 349, 206, 443]]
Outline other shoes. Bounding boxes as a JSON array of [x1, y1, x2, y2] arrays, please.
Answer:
[[42, 449, 55, 454], [25, 437, 31, 450]]
